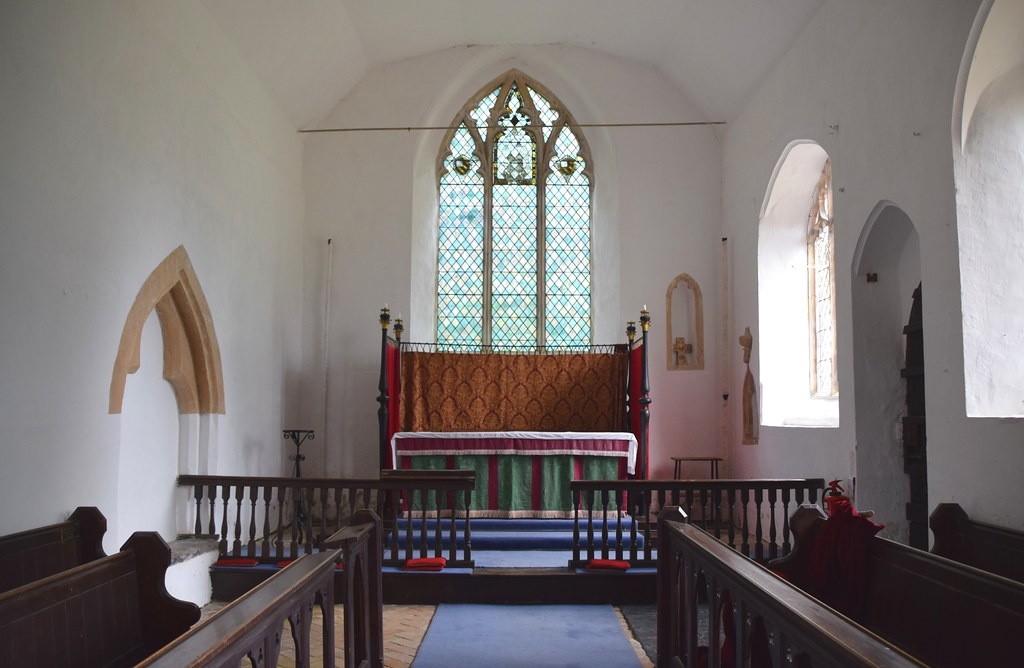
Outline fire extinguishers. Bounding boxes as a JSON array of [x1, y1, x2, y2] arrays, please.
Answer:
[[822, 477, 844, 514]]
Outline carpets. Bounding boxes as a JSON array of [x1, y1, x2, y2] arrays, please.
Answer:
[[211, 519, 783, 577], [409, 604, 643, 667], [618, 599, 743, 664]]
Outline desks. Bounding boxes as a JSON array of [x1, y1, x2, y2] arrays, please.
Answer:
[[670, 455, 723, 509], [391, 431, 637, 520]]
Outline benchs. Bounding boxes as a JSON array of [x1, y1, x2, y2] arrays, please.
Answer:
[[0, 506, 202, 668], [762, 502, 1024, 668]]
